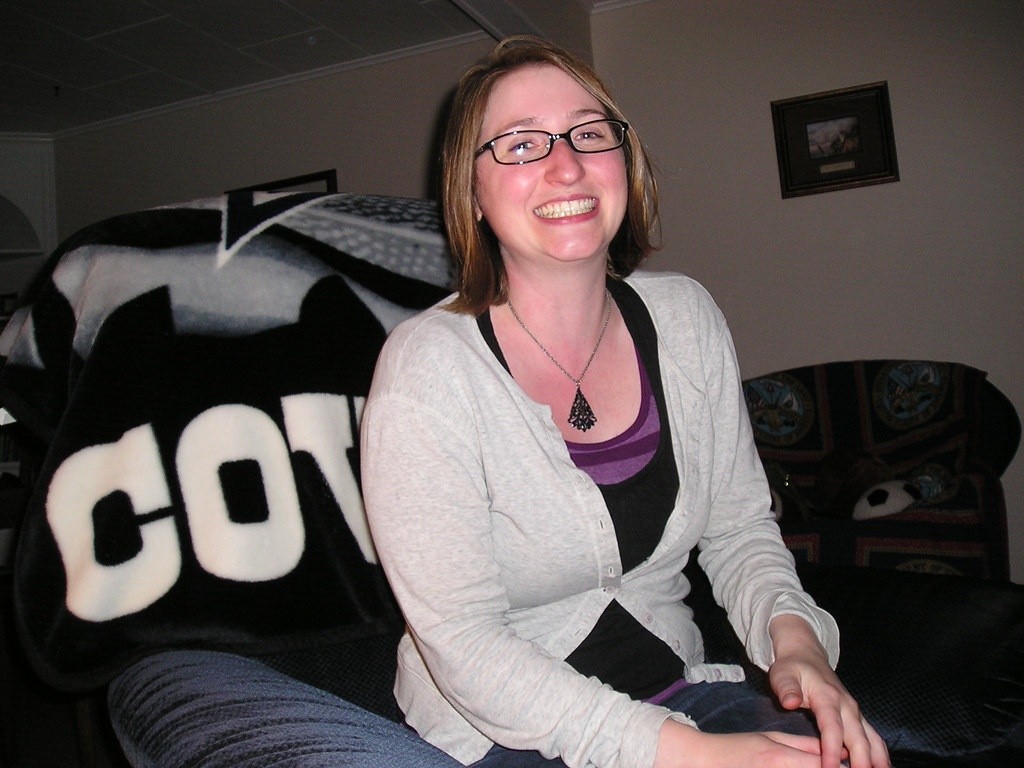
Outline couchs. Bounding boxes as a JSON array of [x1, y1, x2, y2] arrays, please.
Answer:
[[1, 186, 1023, 767]]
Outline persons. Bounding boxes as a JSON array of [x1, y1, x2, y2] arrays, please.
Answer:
[[359, 37, 893, 768]]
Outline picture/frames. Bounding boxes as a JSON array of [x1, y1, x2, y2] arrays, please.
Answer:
[[770, 81, 899, 199]]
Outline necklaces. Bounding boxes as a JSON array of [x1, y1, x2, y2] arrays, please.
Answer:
[[505, 289, 613, 431]]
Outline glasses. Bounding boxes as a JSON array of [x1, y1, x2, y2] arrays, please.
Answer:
[[474, 119, 629, 164]]
